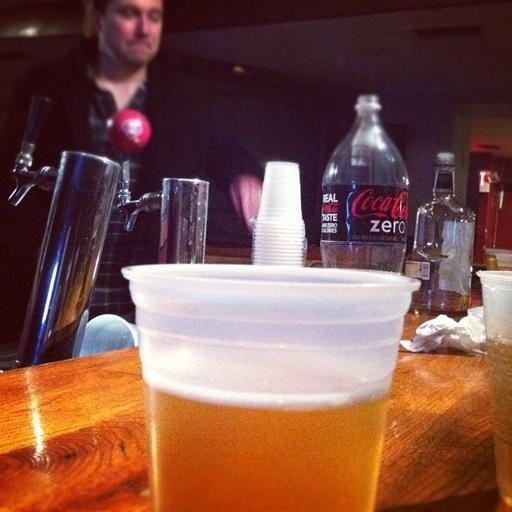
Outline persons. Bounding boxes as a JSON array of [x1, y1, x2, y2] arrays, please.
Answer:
[[10, 1, 262, 228]]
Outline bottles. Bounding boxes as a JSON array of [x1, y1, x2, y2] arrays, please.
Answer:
[[320, 95, 412, 274], [408, 151, 477, 320]]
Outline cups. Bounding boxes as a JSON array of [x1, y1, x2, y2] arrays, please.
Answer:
[[80, 312, 140, 360], [252, 160, 305, 265]]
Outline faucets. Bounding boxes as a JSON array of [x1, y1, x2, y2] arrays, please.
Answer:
[[6, 154, 54, 207], [117, 190, 160, 234]]
